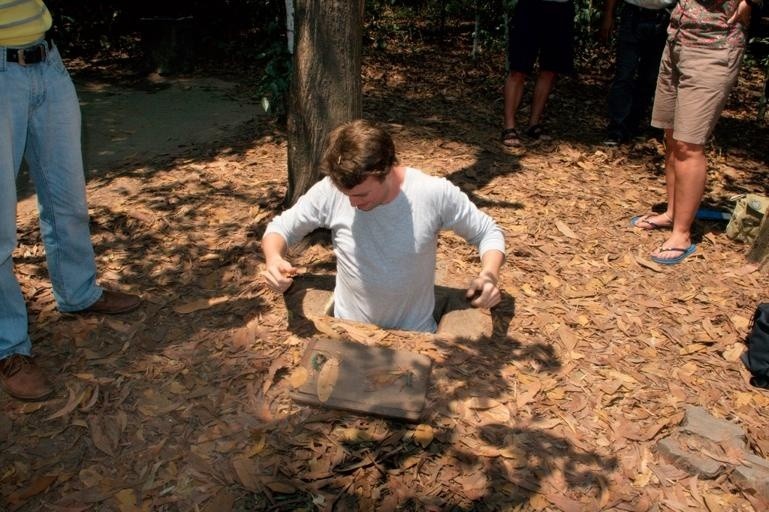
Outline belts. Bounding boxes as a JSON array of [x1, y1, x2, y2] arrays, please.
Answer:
[[6, 38, 52, 65]]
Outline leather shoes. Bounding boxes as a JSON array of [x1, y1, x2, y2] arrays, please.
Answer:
[[87, 290, 139, 314], [0, 353, 54, 401]]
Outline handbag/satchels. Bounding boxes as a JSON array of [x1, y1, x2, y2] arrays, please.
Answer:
[[741, 302, 769, 377]]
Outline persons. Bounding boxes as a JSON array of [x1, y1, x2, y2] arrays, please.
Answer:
[[498, 0, 576, 152], [598, 1, 683, 149], [259, 117, 507, 333], [1, 1, 143, 404], [626, 1, 762, 267]]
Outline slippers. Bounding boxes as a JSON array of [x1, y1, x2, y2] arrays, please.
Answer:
[[501, 128, 523, 147], [651, 241, 696, 264], [631, 214, 658, 228], [522, 124, 547, 140]]
